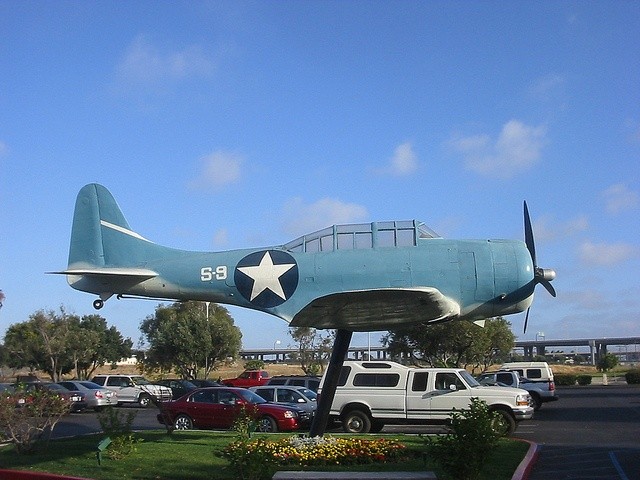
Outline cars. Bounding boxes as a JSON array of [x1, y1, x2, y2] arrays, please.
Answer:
[[152, 380, 203, 400], [243, 387, 344, 428], [157, 386, 302, 434], [58, 381, 117, 412], [9, 381, 86, 418]]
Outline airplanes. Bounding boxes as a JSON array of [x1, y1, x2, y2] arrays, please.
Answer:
[[43, 183, 556, 334]]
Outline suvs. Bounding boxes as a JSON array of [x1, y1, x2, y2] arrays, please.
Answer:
[[265, 376, 324, 388], [500, 362, 553, 378], [456, 371, 559, 410], [90, 375, 174, 409], [316, 360, 534, 438], [221, 371, 271, 386]]
